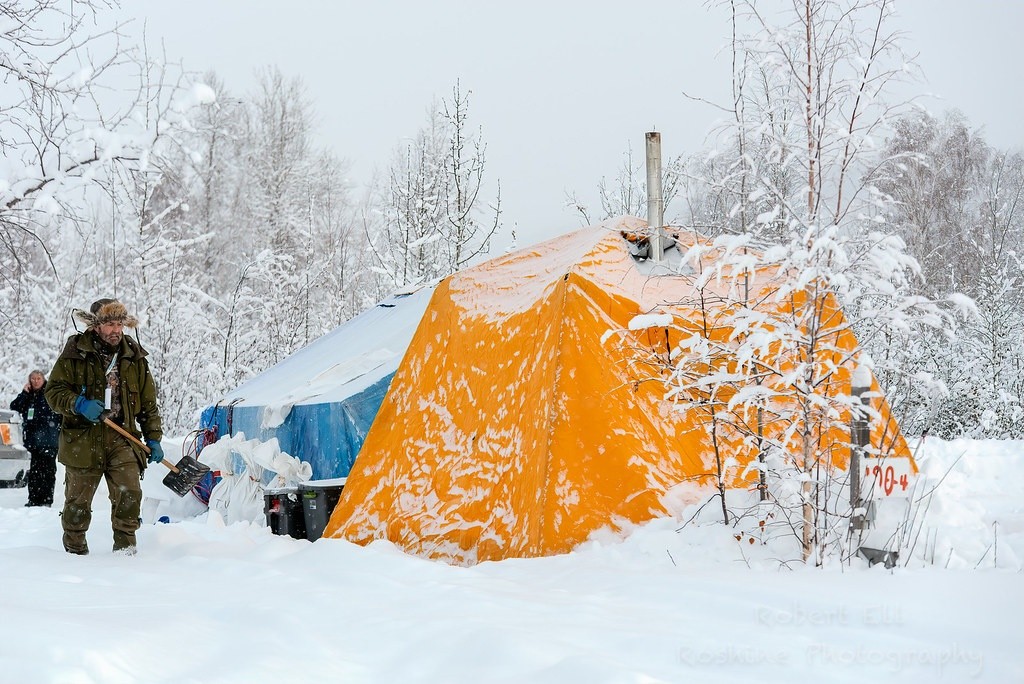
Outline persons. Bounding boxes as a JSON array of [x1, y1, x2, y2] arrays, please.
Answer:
[[10, 370, 63, 508], [43, 299, 165, 555]]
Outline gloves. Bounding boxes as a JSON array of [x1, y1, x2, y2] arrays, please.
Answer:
[[145, 441, 164, 464], [74, 396, 106, 420]]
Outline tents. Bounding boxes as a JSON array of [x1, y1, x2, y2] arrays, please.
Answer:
[[198, 217, 914, 568]]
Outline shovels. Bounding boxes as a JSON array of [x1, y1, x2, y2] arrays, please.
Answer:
[[99, 412, 210, 498]]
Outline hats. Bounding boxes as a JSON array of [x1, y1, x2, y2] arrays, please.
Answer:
[[71, 298, 143, 351]]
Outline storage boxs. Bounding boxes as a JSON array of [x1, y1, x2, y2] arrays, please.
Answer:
[[298, 477, 348, 543], [263, 487, 307, 540]]
